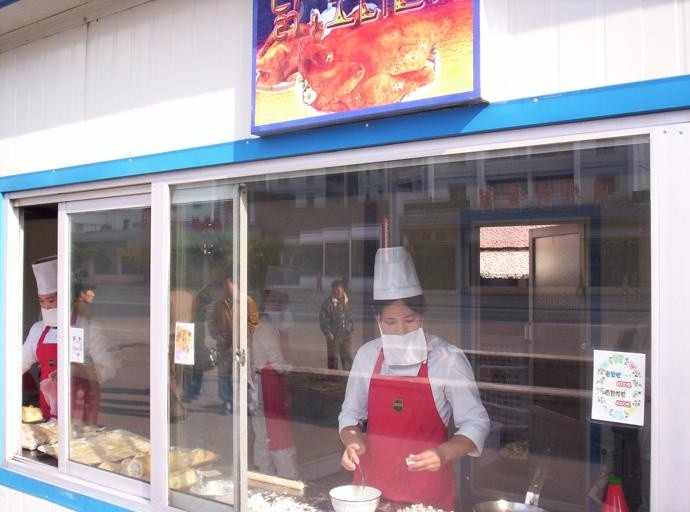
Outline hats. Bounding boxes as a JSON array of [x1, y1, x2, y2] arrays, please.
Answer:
[[32, 255, 57, 296], [373, 247, 423, 300], [264, 266, 285, 293], [73, 276, 97, 288]]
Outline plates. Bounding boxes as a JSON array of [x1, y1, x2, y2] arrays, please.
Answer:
[[22, 417, 48, 425]]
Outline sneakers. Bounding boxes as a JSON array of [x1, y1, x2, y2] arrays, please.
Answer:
[[223, 404, 231, 414], [181, 392, 199, 403]]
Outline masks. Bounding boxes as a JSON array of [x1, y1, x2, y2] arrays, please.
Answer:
[[264, 309, 293, 331], [40, 308, 57, 328], [375, 319, 428, 367]]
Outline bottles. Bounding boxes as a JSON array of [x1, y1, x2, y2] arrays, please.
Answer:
[[601, 476, 629, 511]]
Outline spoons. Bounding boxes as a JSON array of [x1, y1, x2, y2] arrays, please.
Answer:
[[358, 463, 367, 490]]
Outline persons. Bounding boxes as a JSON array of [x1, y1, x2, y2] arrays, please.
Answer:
[[248, 289, 305, 481], [22, 290, 128, 421], [170, 283, 193, 423], [184, 285, 215, 402], [320, 279, 356, 371], [337, 295, 490, 512], [70, 276, 108, 431], [207, 275, 259, 413]]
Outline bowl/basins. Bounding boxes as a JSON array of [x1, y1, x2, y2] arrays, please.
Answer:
[[329, 485, 382, 512], [473, 500, 546, 511]]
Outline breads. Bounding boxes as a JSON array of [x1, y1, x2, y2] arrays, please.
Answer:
[[21, 402, 215, 491]]
